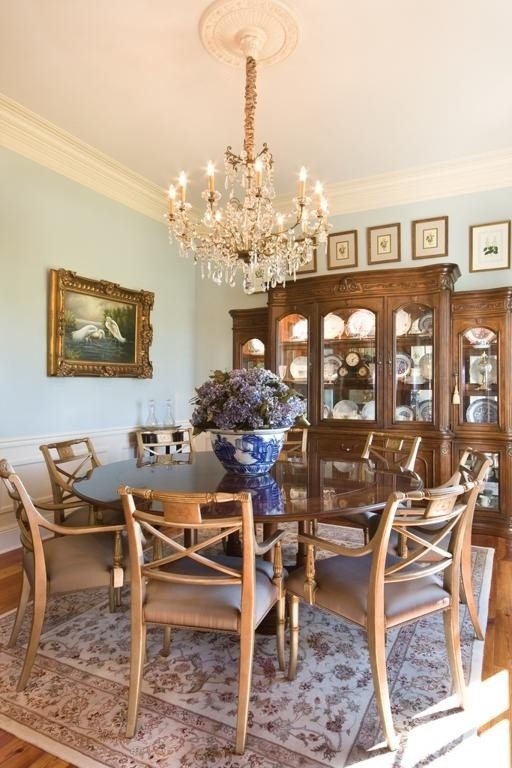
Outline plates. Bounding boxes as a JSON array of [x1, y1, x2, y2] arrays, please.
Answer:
[[396, 352, 414, 379], [290, 356, 307, 379], [464, 326, 497, 345], [333, 399, 358, 420], [332, 461, 355, 473], [282, 486, 340, 508], [292, 319, 309, 341], [418, 353, 432, 380], [395, 308, 412, 336], [395, 400, 431, 422], [323, 313, 345, 339], [361, 400, 374, 421], [465, 399, 497, 424], [324, 353, 343, 382], [345, 308, 375, 337], [417, 312, 432, 333]]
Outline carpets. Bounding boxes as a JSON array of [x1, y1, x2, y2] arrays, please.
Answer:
[[0, 518, 498, 768]]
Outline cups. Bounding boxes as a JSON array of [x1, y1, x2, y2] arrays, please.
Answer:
[[323, 405, 330, 418], [478, 495, 490, 507], [278, 365, 286, 378]]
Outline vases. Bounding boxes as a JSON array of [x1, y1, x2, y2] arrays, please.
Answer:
[[215, 472, 286, 515], [204, 428, 288, 477]]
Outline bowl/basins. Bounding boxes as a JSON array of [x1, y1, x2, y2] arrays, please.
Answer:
[[345, 411, 364, 421]]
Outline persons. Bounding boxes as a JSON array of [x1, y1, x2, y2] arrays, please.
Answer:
[[69, 314, 127, 350]]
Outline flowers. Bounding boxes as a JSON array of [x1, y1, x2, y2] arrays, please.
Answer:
[[181, 369, 312, 433]]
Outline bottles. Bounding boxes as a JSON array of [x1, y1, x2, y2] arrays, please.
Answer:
[[145, 398, 176, 426]]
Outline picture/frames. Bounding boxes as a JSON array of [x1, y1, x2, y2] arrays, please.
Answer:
[[326, 230, 358, 270], [410, 216, 449, 260], [45, 266, 154, 379], [293, 237, 319, 276], [470, 220, 508, 273], [365, 222, 402, 266]]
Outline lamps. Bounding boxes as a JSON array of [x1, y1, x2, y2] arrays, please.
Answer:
[[161, 0, 332, 293]]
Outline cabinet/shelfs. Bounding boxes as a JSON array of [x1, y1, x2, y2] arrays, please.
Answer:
[[311, 425, 383, 527], [383, 431, 452, 518], [452, 437, 512, 541], [452, 285, 512, 437], [314, 262, 464, 434], [265, 278, 312, 429]]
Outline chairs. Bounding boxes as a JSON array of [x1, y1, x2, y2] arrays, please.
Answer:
[[1, 457, 173, 691], [134, 426, 197, 456], [365, 448, 493, 640], [135, 454, 198, 468], [284, 485, 483, 749], [332, 430, 422, 526], [118, 483, 280, 753], [37, 436, 165, 562]]
